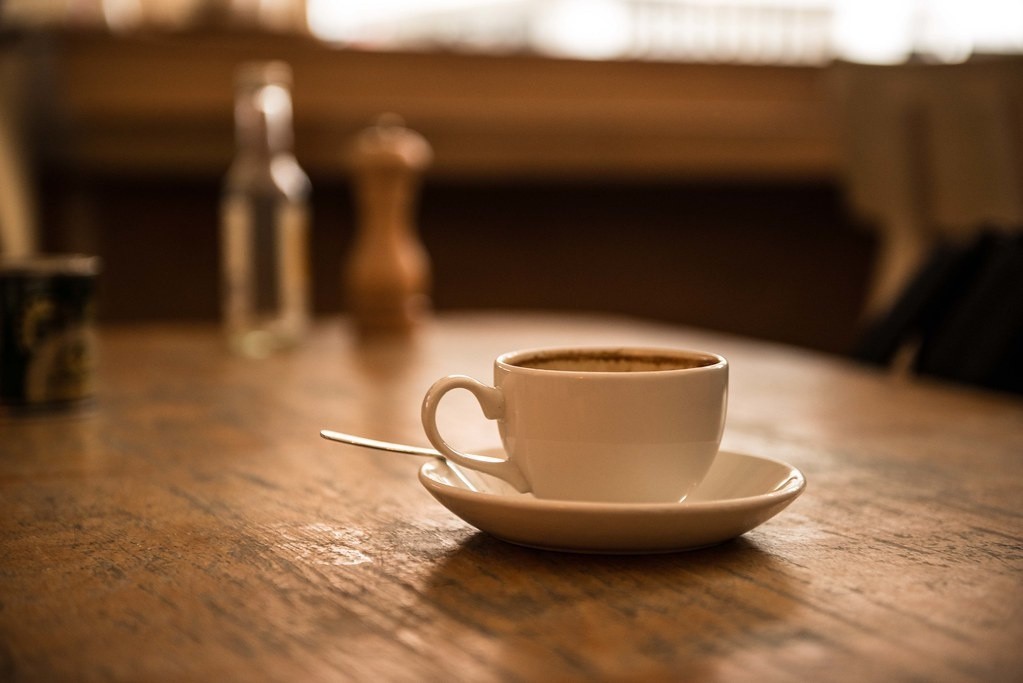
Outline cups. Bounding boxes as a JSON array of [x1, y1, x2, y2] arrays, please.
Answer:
[[421, 345, 729, 504], [0, 254, 99, 403]]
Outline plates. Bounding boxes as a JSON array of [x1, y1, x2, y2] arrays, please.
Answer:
[[419, 449, 807, 553]]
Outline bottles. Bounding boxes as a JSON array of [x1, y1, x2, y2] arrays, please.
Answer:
[[350, 114, 427, 338], [222, 54, 305, 363]]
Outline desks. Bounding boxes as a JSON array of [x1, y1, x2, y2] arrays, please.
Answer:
[[0, 308, 1023, 683]]
